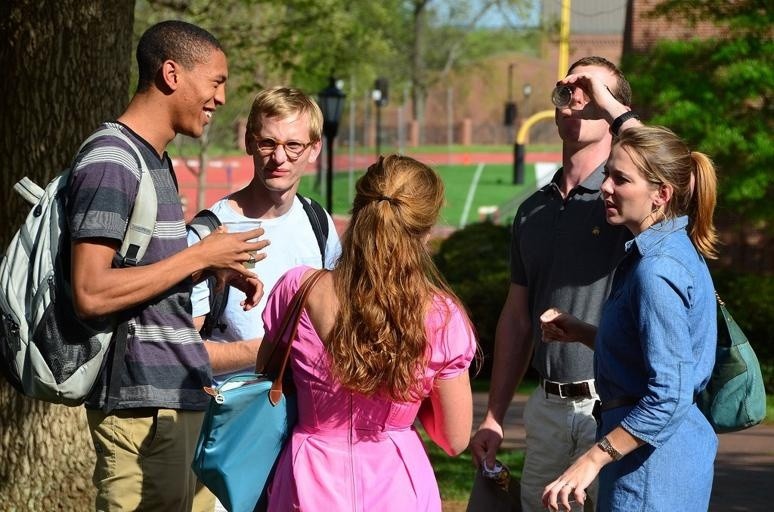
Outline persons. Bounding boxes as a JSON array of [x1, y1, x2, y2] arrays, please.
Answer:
[[471, 56, 646, 511], [65, 20, 269, 512], [540, 126, 720, 512], [185, 85, 343, 390], [254, 154, 479, 512]]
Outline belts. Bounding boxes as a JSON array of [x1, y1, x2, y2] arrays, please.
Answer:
[[536, 377, 594, 399], [592, 396, 640, 419]]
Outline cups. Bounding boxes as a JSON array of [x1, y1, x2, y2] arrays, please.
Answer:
[[550, 82, 587, 113], [223, 220, 264, 270]]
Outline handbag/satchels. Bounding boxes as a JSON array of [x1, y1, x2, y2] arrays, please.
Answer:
[[188, 378, 288, 511], [696, 304, 766, 433]]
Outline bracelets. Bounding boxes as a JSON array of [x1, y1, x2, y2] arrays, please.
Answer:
[[609, 111, 640, 136]]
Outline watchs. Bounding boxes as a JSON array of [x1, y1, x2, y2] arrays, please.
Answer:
[[598, 435, 624, 461]]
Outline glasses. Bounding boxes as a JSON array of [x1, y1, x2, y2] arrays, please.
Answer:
[[245, 124, 320, 161]]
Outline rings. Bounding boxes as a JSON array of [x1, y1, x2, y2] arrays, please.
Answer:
[[248, 253, 256, 264]]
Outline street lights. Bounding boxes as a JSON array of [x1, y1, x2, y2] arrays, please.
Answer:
[[370, 79, 384, 161], [521, 83, 532, 122], [315, 66, 346, 217]]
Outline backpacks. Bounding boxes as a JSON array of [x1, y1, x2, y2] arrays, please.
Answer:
[[0, 130, 158, 405]]
